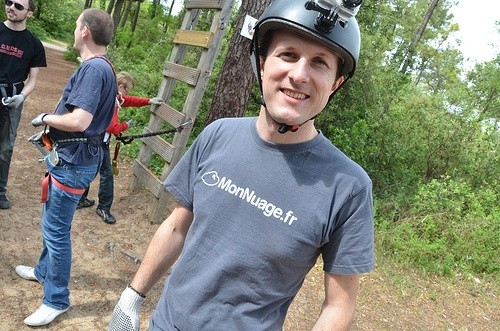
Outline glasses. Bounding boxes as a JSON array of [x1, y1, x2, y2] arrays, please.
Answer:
[[124, 87, 128, 93], [5, 0, 29, 10]]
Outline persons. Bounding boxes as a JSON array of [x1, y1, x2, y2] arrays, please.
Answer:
[[107, 0, 377, 331], [0, 0, 48, 211], [76, 71, 164, 223], [16, 8, 119, 324]]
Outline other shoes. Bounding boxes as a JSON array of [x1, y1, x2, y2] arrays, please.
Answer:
[[0, 195, 11, 210]]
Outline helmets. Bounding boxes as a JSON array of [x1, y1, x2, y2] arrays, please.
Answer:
[[249, 0, 365, 78]]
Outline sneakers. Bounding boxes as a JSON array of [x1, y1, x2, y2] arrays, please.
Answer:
[[15, 264, 38, 281], [23, 304, 71, 327], [96, 208, 116, 224], [76, 198, 96, 209]]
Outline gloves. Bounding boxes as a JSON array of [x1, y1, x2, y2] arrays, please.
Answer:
[[30, 112, 48, 127], [4, 93, 25, 109], [149, 96, 165, 105], [108, 284, 147, 331], [126, 118, 136, 128]]
